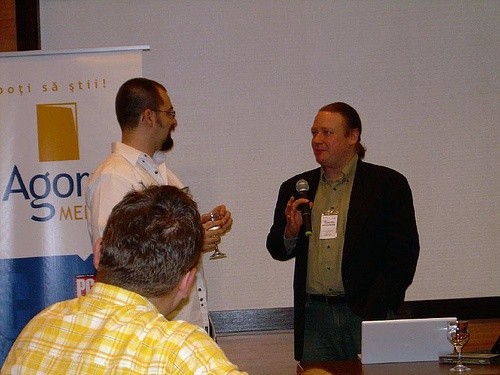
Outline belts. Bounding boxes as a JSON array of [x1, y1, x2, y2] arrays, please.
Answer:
[[305, 296, 347, 305]]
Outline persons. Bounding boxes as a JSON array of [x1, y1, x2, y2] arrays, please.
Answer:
[[266, 102, 420, 362], [91, 78, 233, 356], [0, 186, 248, 375]]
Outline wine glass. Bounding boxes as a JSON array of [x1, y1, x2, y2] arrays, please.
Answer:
[[447, 321, 472, 371], [202, 208, 227, 260]]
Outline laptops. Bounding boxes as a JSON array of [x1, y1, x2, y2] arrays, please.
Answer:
[[356, 316, 458, 366]]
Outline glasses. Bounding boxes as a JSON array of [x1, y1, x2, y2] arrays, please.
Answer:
[[141, 109, 176, 122]]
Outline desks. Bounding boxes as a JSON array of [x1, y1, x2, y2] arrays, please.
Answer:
[[296, 319, 500, 375]]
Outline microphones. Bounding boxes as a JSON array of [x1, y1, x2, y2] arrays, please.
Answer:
[[296, 178, 313, 236]]
